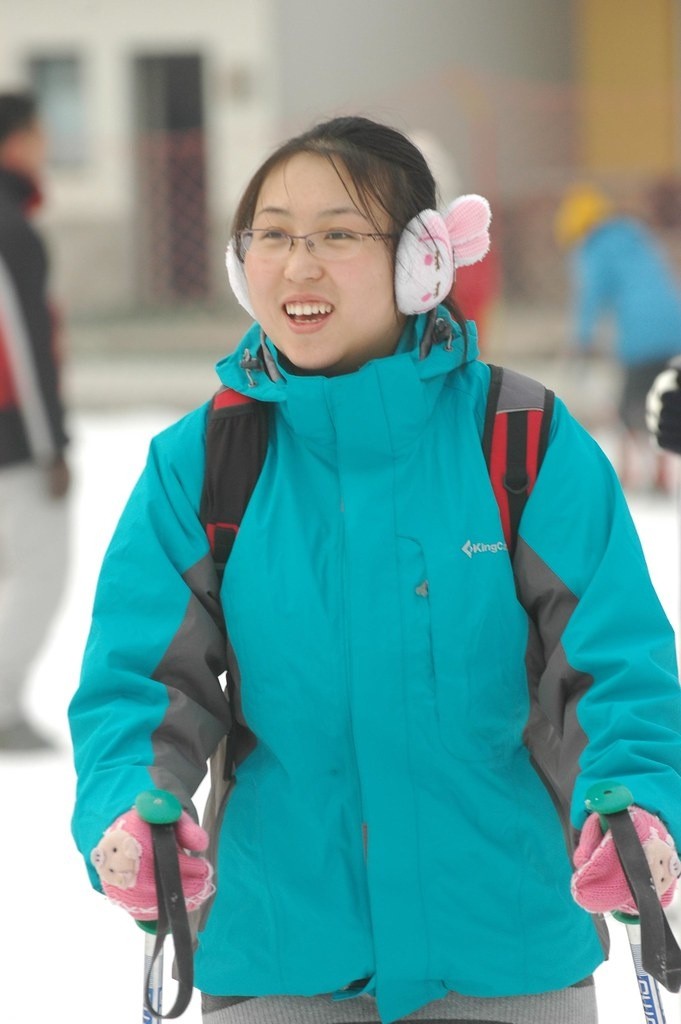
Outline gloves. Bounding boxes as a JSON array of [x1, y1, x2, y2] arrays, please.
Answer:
[[91, 805, 217, 921], [570, 805, 681, 916]]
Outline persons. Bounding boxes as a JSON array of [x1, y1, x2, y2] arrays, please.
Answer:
[[549, 187, 681, 502], [0, 91, 74, 755], [69, 115, 681, 1024]]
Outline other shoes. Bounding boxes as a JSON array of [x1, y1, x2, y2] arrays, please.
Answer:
[[0, 722, 52, 751]]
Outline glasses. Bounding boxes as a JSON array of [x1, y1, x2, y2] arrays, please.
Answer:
[[240, 229, 393, 262]]
